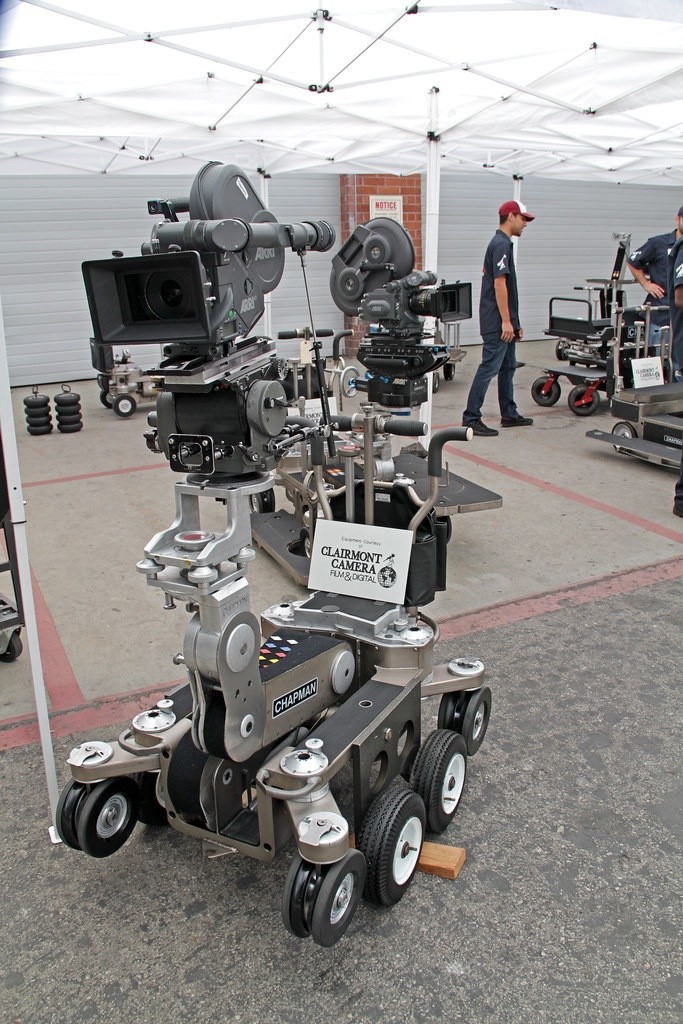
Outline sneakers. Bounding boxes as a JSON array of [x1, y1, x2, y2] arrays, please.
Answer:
[[501, 414, 533, 427], [466, 420, 499, 436]]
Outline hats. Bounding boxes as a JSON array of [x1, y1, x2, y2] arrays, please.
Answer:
[[498, 200, 535, 222], [678, 206, 683, 215]]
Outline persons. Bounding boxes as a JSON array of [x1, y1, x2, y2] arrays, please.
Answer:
[[627, 206, 683, 327], [462, 199, 535, 435], [666, 237, 683, 518]]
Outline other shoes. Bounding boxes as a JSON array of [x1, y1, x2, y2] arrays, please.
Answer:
[[672, 506, 683, 517]]
